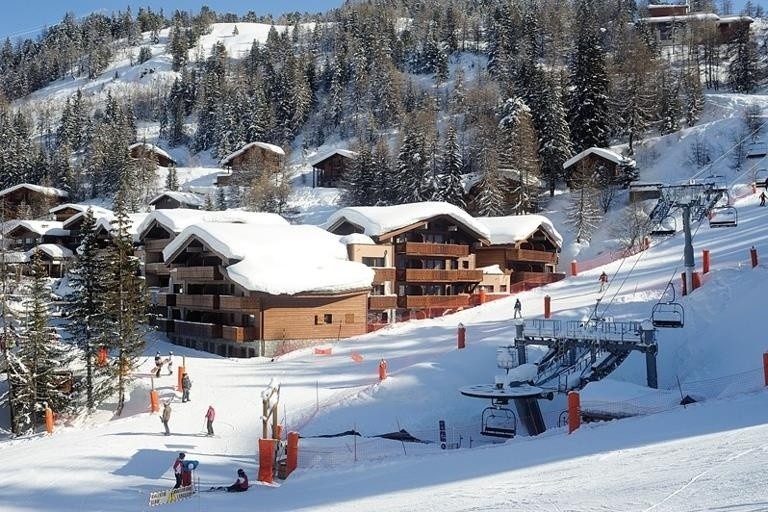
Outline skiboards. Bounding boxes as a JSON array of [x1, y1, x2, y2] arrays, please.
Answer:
[[198, 487, 226, 492], [193, 432, 218, 438]]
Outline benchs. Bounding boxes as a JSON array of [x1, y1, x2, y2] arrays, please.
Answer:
[[148, 483, 196, 507]]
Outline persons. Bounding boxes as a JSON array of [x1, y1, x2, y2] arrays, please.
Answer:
[[216, 468, 249, 492], [171, 452, 186, 489], [598, 270, 609, 292], [181, 372, 191, 403], [205, 405, 216, 435], [161, 401, 171, 436], [166, 350, 174, 375], [513, 297, 523, 319], [757, 191, 767, 207], [154, 350, 163, 378], [185, 375, 191, 401]]
